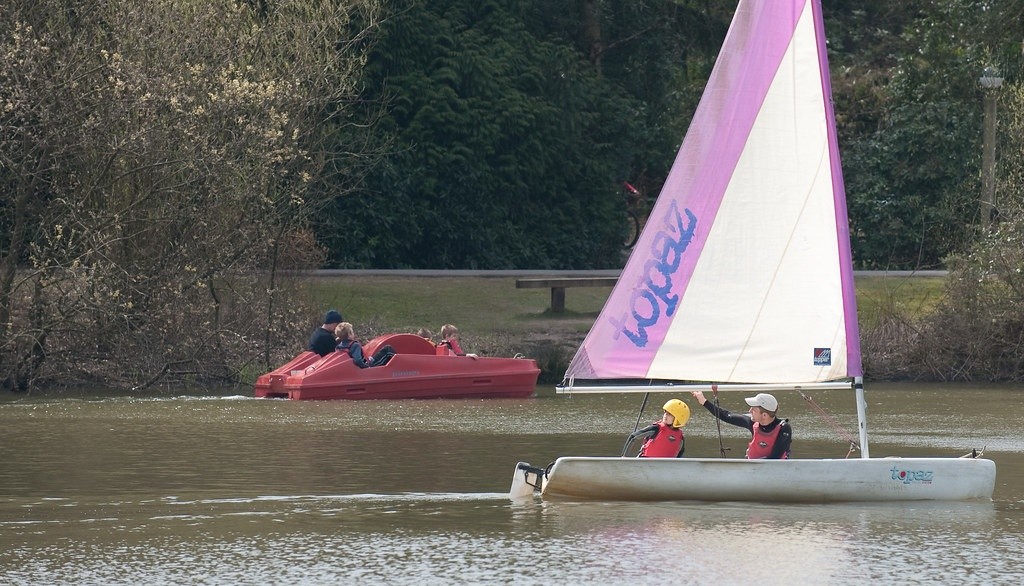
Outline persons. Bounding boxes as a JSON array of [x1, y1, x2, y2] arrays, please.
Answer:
[[691, 389, 793, 460], [416, 327, 437, 348], [308, 310, 344, 357], [333, 321, 396, 369], [438, 323, 479, 361], [621, 399, 690, 459]]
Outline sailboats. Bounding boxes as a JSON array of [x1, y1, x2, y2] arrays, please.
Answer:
[[509, 0, 996, 504]]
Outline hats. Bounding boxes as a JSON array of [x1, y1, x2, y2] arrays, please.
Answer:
[[323, 310, 343, 324], [745, 393, 778, 412]]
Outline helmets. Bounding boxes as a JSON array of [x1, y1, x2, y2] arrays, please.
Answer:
[[662, 398, 690, 428]]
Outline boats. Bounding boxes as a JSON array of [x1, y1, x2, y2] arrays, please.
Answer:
[[255, 330, 543, 400]]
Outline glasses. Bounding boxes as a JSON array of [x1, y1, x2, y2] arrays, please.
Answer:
[[664, 410, 673, 416]]
